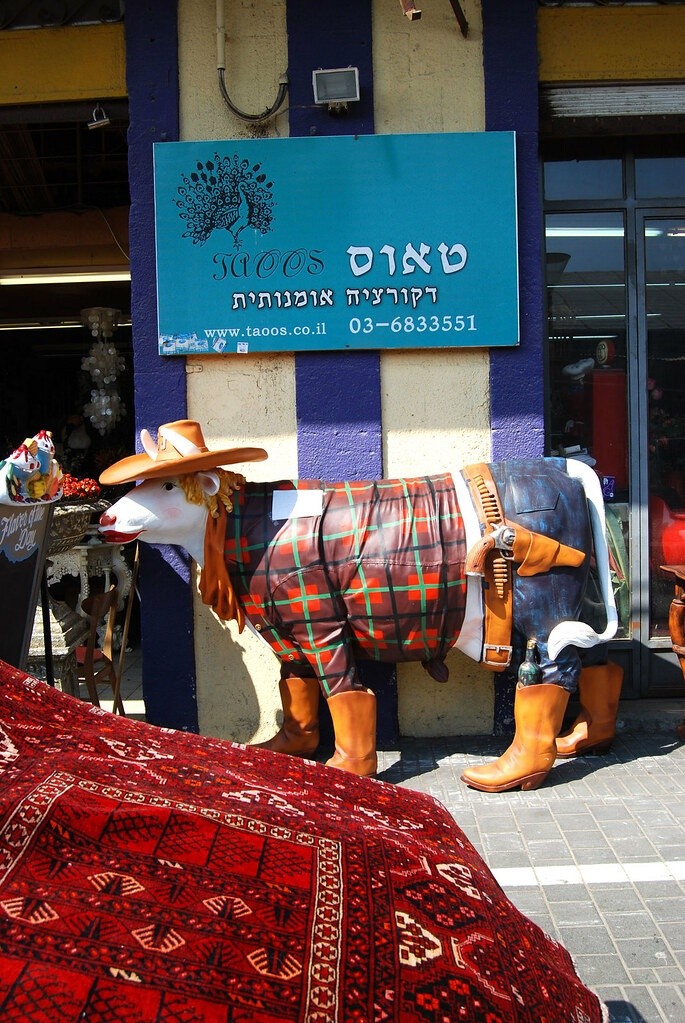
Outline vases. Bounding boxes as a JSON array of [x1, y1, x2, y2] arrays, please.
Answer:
[[44, 500, 111, 559]]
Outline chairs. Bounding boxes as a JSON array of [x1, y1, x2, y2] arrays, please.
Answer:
[[73, 584, 127, 718]]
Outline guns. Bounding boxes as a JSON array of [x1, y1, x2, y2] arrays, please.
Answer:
[[464, 523, 523, 576]]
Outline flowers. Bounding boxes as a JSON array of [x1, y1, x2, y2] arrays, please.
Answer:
[[57, 471, 98, 504]]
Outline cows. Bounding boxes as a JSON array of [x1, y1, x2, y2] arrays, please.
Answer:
[[96, 455, 623, 786]]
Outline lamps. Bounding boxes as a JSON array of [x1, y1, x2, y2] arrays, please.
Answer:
[[312, 67, 361, 112]]
